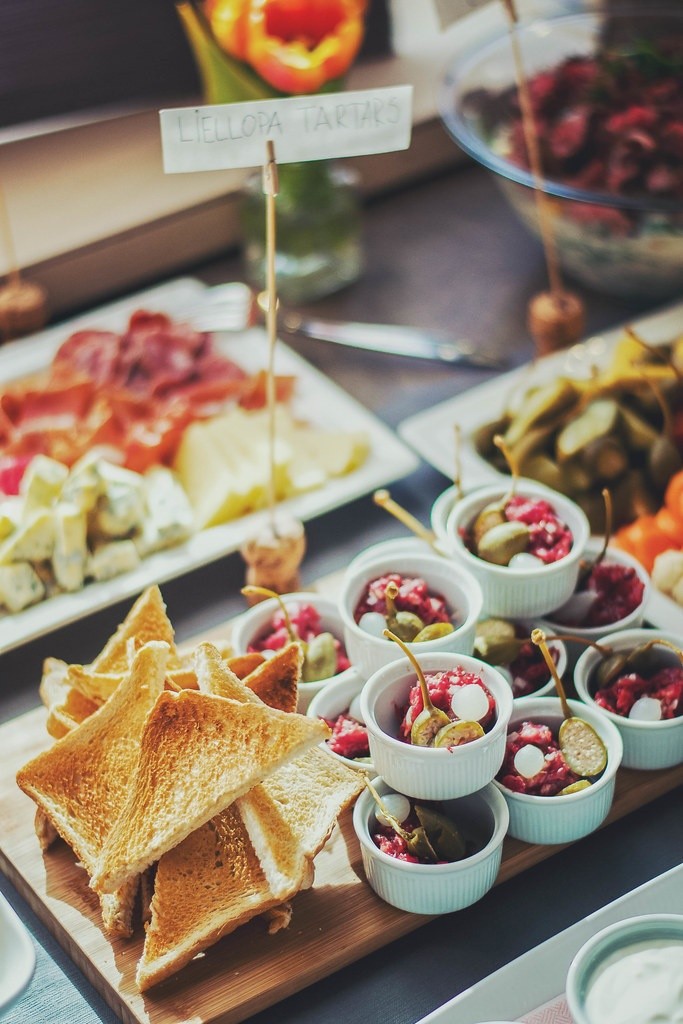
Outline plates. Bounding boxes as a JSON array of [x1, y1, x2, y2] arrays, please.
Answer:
[[396, 301, 683, 645], [0, 277, 422, 661]]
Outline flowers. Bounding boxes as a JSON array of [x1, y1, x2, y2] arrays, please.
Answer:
[[176, 0, 368, 218]]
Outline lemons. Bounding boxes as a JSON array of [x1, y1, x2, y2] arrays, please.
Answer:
[[176, 401, 372, 525]]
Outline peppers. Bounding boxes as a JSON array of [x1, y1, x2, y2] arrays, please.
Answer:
[[497, 38, 682, 246]]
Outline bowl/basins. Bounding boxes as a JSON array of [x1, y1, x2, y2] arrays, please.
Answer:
[[437, 7, 682, 301], [231, 473, 683, 918], [566, 913, 683, 1024]]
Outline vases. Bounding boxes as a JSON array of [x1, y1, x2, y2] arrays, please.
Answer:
[[241, 163, 367, 306]]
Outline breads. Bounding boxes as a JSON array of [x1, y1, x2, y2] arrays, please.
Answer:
[[12, 582, 368, 995]]
